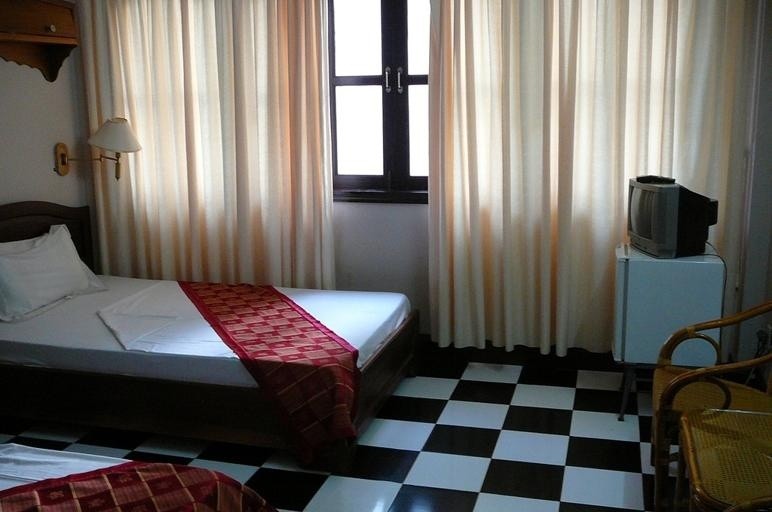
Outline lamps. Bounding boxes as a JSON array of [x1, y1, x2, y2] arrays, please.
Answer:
[[54, 117, 139, 181]]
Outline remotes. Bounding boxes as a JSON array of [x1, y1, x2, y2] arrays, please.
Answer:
[[636, 175, 675, 184]]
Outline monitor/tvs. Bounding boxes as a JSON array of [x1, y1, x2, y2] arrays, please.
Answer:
[[626, 178, 718, 259]]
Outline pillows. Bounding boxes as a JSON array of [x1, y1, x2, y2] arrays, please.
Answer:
[[0, 225, 111, 324]]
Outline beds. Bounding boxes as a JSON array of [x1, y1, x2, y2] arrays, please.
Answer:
[[0, 443, 279, 512], [0, 201, 420, 457]]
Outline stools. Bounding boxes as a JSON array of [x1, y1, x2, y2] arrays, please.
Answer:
[[675, 408, 772, 512]]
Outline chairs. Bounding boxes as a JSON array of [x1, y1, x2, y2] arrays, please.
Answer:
[[650, 301, 772, 506]]
